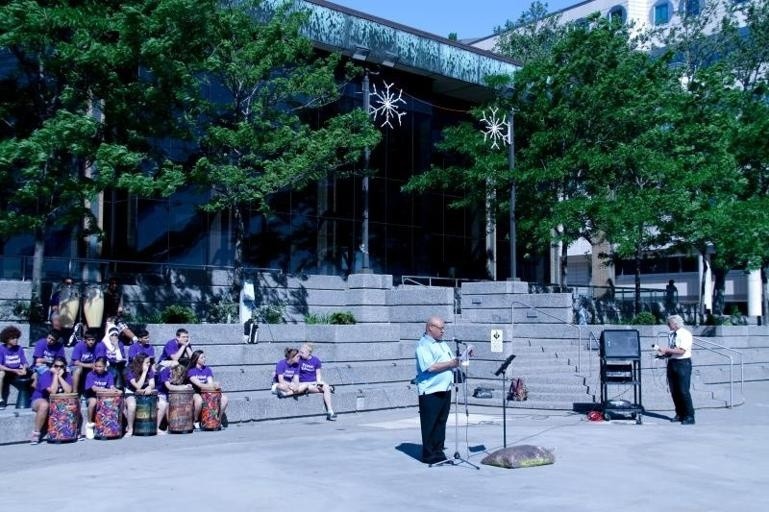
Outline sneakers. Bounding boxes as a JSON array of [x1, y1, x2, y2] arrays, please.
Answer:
[[77, 433, 85, 441], [329, 385, 334, 391], [30, 432, 41, 445], [193, 421, 201, 429], [85, 423, 96, 439], [328, 411, 338, 421], [0, 400, 6, 409]]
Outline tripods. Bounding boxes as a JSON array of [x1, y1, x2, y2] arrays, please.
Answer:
[[428, 387, 481, 470]]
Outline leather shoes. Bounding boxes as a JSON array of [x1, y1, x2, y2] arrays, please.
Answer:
[[423, 457, 453, 465], [682, 419, 695, 424], [672, 417, 682, 422]]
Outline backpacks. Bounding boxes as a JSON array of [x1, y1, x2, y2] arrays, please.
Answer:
[[509, 379, 528, 401]]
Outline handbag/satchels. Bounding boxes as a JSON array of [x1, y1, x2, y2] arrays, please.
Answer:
[[475, 387, 492, 398]]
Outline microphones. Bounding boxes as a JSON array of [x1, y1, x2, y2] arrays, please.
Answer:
[[452, 338, 464, 344], [651, 344, 668, 357]]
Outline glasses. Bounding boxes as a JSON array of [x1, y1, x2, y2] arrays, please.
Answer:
[[54, 365, 65, 368], [66, 282, 73, 285]]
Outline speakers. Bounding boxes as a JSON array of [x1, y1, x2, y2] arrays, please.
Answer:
[[599, 329, 641, 358]]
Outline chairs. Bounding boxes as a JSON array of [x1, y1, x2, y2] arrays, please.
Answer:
[[600, 329, 644, 424]]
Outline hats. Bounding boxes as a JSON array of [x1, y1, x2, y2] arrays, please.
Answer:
[[110, 328, 119, 335]]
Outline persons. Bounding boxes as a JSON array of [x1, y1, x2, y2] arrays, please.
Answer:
[[49, 276, 80, 333], [416, 316, 474, 465], [100, 326, 125, 384], [600, 277, 615, 304], [666, 278, 678, 309], [128, 330, 155, 366], [299, 345, 338, 422], [84, 357, 125, 440], [31, 357, 72, 445], [102, 278, 139, 345], [157, 328, 193, 368], [32, 328, 71, 371], [121, 352, 167, 439], [157, 364, 193, 432], [69, 331, 107, 402], [0, 325, 30, 409], [270, 348, 308, 399], [186, 349, 229, 431], [651, 313, 695, 426]]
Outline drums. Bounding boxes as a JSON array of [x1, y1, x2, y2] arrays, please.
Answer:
[[134, 389, 159, 436], [47, 391, 79, 442], [167, 389, 196, 434], [196, 386, 225, 430], [95, 390, 124, 441]]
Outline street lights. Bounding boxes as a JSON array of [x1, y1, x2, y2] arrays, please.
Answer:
[[502, 86, 538, 281], [350, 45, 400, 274]]
